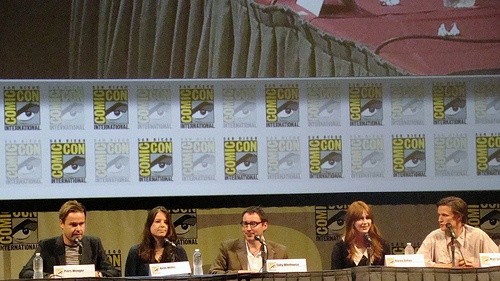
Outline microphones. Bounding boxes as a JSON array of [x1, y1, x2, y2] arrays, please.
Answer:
[[163, 238, 176, 247], [446, 223, 455, 237], [364, 233, 371, 241], [255, 235, 266, 245], [74, 238, 83, 248]]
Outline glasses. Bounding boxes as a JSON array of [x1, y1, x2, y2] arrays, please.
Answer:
[[240, 220, 262, 228]]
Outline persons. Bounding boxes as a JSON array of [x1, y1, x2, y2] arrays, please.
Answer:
[[331, 201, 390, 269], [125, 206, 189, 276], [18, 200, 121, 278], [416, 197, 498, 268], [208, 206, 288, 276]]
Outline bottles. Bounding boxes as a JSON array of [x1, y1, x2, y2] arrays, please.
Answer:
[[33, 252, 44, 279], [193, 249, 203, 275], [405, 242, 414, 255]]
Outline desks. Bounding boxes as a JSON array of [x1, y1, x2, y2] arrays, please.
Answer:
[[0, 266, 500, 281]]
